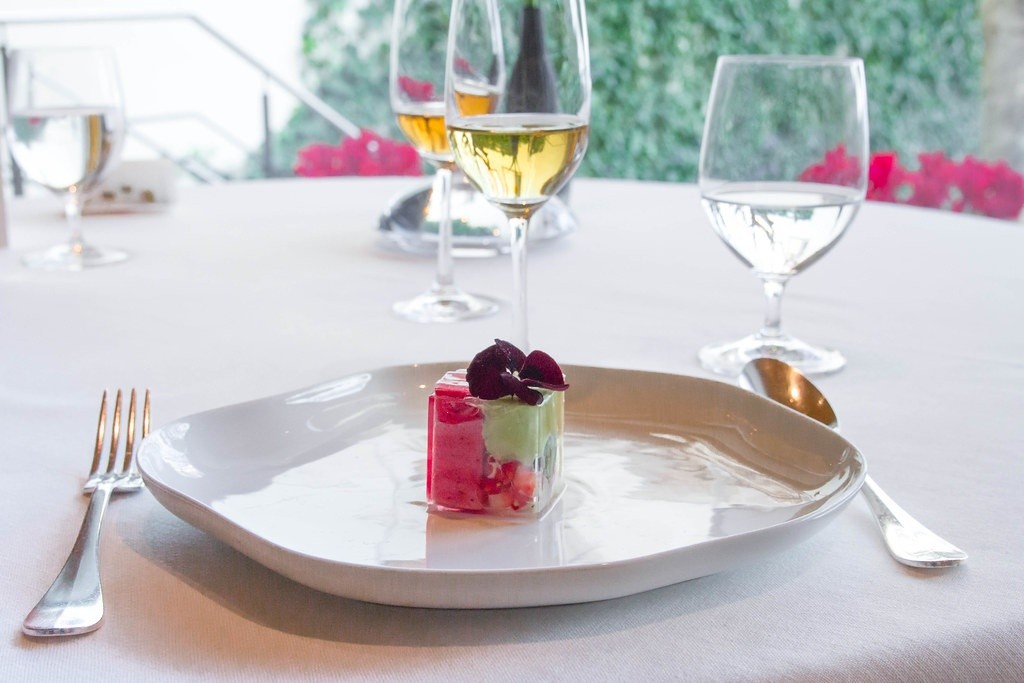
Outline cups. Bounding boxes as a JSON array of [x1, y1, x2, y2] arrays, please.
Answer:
[[425, 367, 564, 521]]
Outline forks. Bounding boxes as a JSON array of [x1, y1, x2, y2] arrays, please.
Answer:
[[24, 389, 151, 639]]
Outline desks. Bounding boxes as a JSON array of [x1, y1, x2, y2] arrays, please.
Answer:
[[1, 175, 1024, 683]]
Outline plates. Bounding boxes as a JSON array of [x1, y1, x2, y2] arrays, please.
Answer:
[[382, 180, 574, 254], [132, 361, 866, 612]]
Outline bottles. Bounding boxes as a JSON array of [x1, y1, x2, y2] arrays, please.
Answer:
[[505, 6, 572, 205]]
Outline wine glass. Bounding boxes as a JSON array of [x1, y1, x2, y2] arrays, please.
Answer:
[[444, 2, 591, 367], [389, 0, 504, 323], [697, 51, 870, 377], [1, 47, 132, 268]]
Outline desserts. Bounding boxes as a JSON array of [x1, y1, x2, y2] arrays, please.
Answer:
[[426, 339, 570, 522]]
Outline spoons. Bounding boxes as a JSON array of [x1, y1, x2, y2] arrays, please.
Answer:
[[738, 354, 970, 569]]
